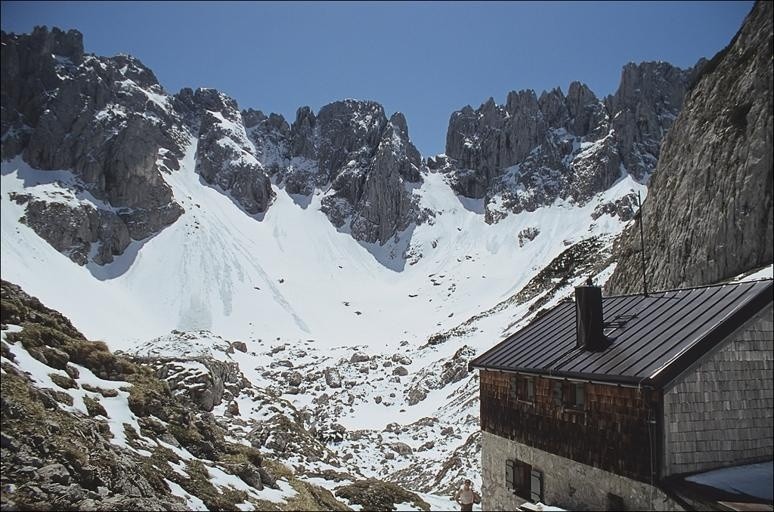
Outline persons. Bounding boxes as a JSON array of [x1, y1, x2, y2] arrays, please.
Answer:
[[455, 479, 474, 512]]
